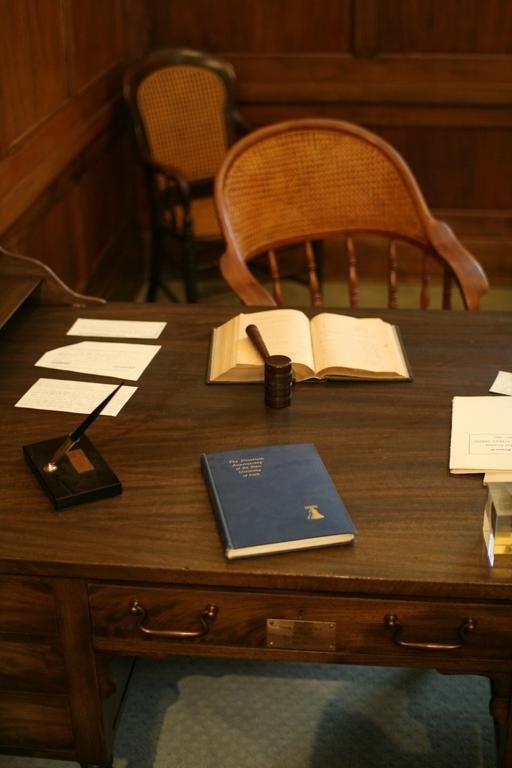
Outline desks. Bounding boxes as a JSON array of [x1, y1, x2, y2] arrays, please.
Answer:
[[0, 248, 511, 768]]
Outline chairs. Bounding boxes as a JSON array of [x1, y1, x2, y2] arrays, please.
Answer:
[[120, 39, 266, 305], [212, 116, 493, 312]]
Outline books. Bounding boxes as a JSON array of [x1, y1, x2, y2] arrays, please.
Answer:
[[449, 394, 511, 487], [200, 443, 358, 562], [205, 308, 413, 384]]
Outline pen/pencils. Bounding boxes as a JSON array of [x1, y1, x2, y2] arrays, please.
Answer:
[[46, 381, 125, 471]]
